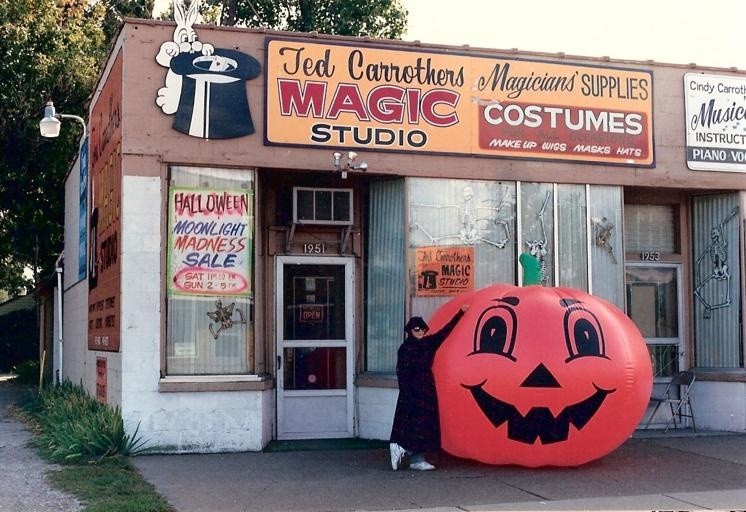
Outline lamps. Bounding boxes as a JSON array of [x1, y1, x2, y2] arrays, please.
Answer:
[[38, 99, 86, 140], [333, 151, 368, 180]]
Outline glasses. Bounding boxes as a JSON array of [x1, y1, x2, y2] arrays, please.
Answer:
[[412, 326, 427, 332]]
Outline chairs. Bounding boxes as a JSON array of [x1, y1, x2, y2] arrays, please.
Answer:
[[645, 370, 697, 434]]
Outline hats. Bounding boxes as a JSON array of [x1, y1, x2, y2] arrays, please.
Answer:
[[404, 317, 428, 334]]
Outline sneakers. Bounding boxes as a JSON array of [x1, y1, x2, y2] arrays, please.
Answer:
[[410, 461, 435, 470], [390, 443, 405, 470]]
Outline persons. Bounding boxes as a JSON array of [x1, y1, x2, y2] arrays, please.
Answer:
[[388, 303, 470, 474]]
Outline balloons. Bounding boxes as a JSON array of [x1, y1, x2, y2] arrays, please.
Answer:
[[417, 252, 655, 467]]
